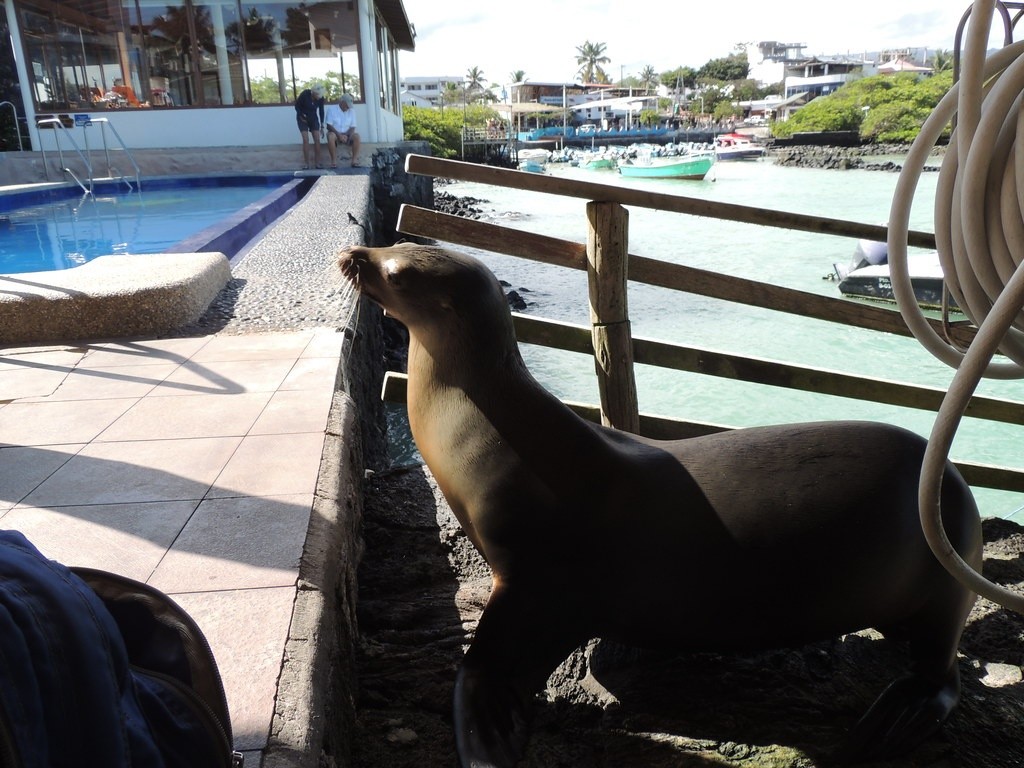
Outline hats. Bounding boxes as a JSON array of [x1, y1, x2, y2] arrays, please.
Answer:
[[340, 93, 354, 109], [310, 84, 323, 99]]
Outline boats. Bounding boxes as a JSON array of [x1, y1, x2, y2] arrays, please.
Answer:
[[549, 142, 715, 163], [509, 148, 551, 163], [516, 160, 546, 174], [714, 136, 764, 161], [616, 155, 714, 180]]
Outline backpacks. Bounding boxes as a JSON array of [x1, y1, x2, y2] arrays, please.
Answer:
[[0, 531, 246, 768]]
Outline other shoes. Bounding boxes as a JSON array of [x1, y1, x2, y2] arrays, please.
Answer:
[[351, 162, 362, 168], [330, 163, 337, 169], [302, 164, 309, 170], [315, 162, 324, 169]]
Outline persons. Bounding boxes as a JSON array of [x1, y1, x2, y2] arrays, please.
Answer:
[[326, 93, 361, 168], [295, 83, 325, 169]]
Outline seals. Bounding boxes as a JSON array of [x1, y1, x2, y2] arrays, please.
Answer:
[[324, 239, 987, 768]]
